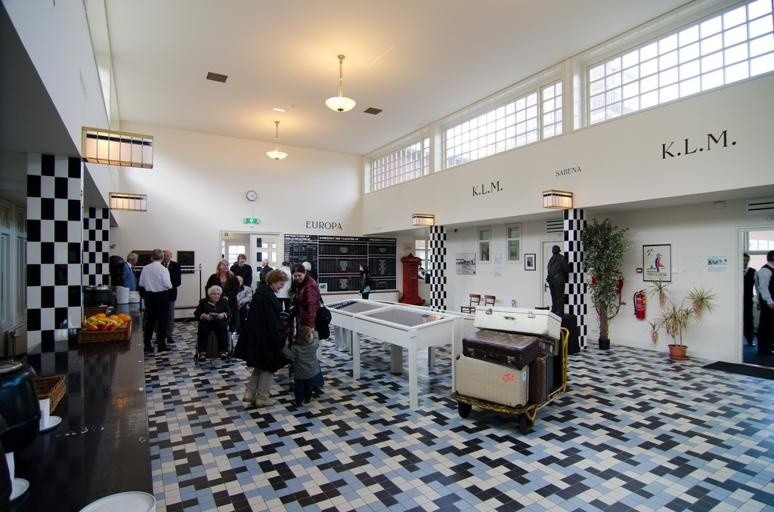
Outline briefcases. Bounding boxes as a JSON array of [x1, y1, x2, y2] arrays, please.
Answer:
[[456, 305, 562, 408]]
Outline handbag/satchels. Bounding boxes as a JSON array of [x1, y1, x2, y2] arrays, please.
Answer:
[[314, 307, 331, 340], [364, 277, 373, 287]]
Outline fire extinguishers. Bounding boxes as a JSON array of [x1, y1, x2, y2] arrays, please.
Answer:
[[632, 288, 646, 319]]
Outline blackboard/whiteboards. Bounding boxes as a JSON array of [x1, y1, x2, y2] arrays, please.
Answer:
[[282, 233, 397, 293]]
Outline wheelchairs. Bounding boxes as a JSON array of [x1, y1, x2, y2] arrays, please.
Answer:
[[195, 315, 232, 362], [240, 292, 254, 333]]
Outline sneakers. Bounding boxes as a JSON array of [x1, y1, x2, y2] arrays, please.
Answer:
[[220, 351, 228, 360], [199, 352, 206, 362]]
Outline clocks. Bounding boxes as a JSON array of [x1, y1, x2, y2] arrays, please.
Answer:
[[246, 190, 257, 201]]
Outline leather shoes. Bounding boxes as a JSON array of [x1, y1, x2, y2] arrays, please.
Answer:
[[243, 391, 255, 402], [167, 337, 174, 343], [158, 346, 171, 352], [144, 347, 154, 352], [256, 399, 277, 407]]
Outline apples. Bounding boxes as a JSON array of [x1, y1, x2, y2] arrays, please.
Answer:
[[85, 317, 129, 331]]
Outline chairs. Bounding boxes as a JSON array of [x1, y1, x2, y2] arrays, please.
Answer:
[[461, 294, 480, 314], [485, 295, 495, 306]]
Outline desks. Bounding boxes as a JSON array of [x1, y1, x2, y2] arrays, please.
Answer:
[[316, 299, 479, 411]]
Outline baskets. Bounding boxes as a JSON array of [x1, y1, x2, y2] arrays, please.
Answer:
[[84, 305, 109, 319], [32, 375, 67, 415], [77, 319, 132, 343]]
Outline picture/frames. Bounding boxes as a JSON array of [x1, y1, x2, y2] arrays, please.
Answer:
[[643, 244, 671, 282], [524, 253, 536, 271]]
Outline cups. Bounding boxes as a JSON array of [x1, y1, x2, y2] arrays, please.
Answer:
[[37, 398, 50, 425], [4, 451, 15, 486]]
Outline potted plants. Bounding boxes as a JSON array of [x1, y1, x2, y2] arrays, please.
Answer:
[[581, 217, 630, 350], [648, 282, 718, 360]]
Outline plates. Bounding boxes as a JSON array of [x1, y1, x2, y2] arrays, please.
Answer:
[[78, 489, 157, 512], [39, 416, 61, 432], [9, 478, 29, 500]]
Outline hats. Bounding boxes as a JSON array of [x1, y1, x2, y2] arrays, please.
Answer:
[[297, 325, 312, 340], [302, 262, 311, 271]]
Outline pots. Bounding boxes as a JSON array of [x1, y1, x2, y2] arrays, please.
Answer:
[[86, 283, 113, 306], [0, 360, 42, 454]]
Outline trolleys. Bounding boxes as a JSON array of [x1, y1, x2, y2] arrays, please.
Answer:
[[451, 326, 568, 434]]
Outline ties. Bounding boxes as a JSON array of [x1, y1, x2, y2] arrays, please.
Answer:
[[164, 264, 167, 268]]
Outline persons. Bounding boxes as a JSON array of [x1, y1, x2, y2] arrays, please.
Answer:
[[139, 248, 171, 354], [548, 245, 568, 317], [194, 254, 324, 409], [359, 264, 372, 298], [744, 254, 757, 347], [154, 250, 181, 343], [122, 253, 140, 325], [755, 251, 774, 366]]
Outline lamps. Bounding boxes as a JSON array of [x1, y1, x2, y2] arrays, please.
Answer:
[[110, 192, 147, 212], [412, 214, 434, 226], [81, 126, 154, 169], [266, 121, 288, 160], [543, 190, 573, 209], [325, 55, 356, 112]]
